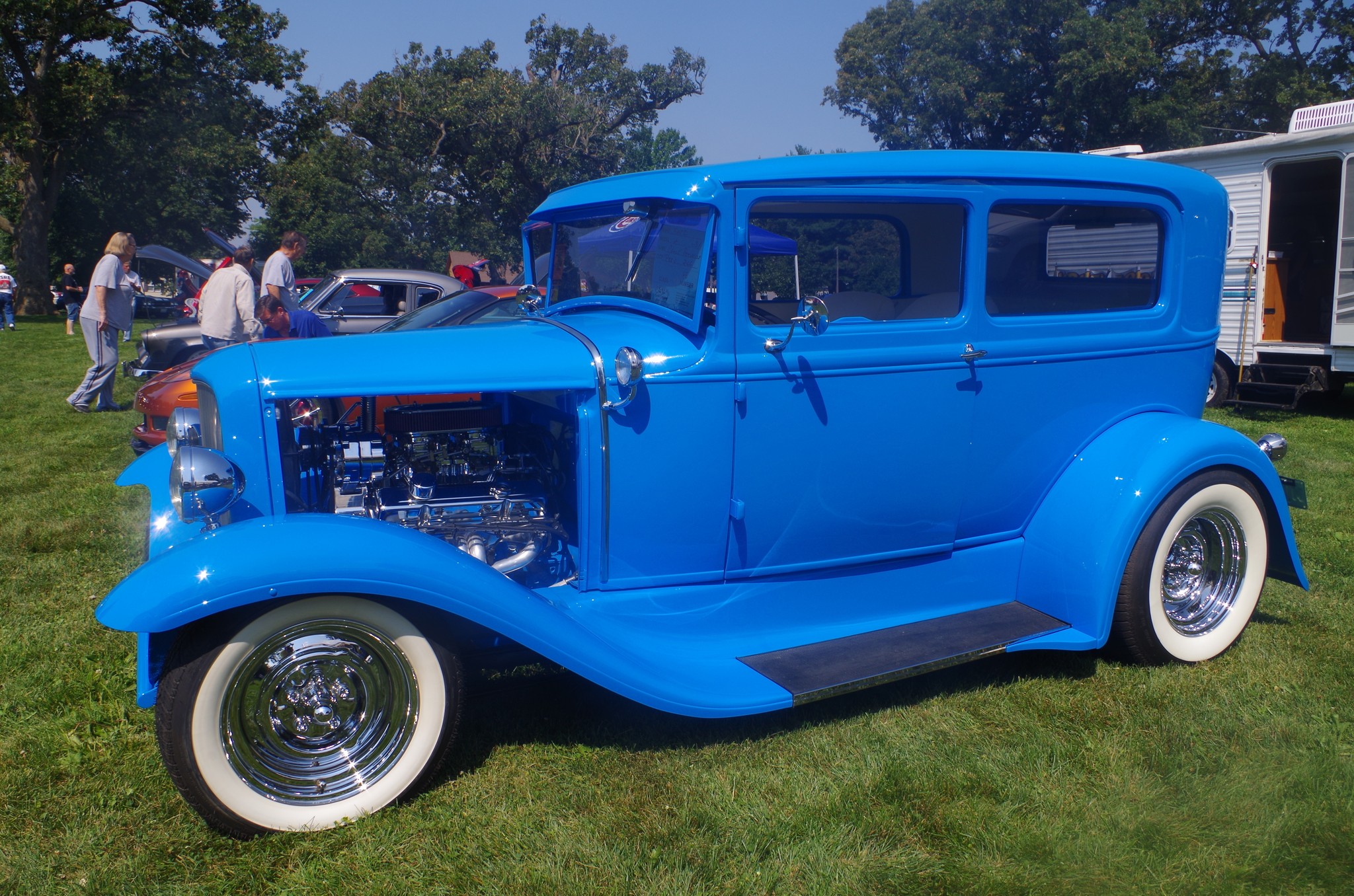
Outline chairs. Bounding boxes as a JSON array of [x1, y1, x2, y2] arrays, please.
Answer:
[[898, 292, 1000, 317], [822, 291, 903, 322]]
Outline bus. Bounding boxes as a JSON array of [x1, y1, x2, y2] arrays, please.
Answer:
[[1046, 97, 1354, 419]]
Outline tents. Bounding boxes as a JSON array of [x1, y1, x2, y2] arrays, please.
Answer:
[[575, 211, 801, 302]]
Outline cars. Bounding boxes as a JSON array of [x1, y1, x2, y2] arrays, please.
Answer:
[[96, 148, 1311, 839]]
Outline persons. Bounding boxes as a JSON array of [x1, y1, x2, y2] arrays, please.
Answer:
[[254, 295, 332, 340], [63, 264, 83, 335], [0, 264, 18, 331], [197, 246, 259, 352], [121, 260, 140, 342], [63, 233, 138, 415], [258, 229, 306, 311]]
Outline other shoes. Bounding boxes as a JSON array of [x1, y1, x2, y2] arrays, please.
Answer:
[[124, 338, 131, 342]]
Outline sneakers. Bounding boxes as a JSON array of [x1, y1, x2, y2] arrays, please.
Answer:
[[1, 323, 16, 331], [64, 397, 124, 413]]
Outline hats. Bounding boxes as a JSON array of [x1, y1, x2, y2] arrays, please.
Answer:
[[0, 264, 6, 270]]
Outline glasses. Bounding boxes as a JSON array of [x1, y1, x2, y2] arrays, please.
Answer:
[[260, 313, 274, 324], [129, 244, 137, 248]]
